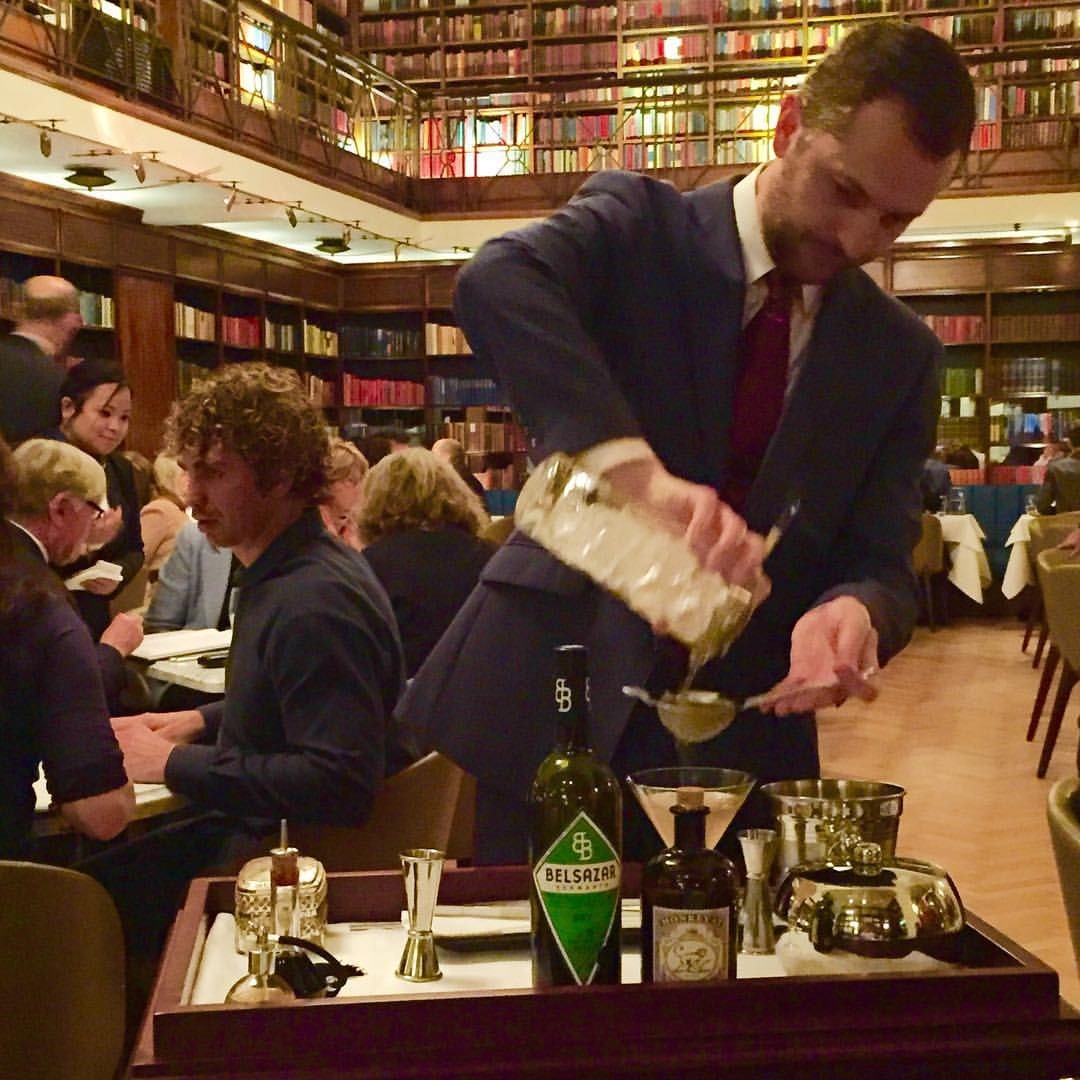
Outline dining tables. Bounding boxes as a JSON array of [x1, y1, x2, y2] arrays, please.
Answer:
[[924, 507, 985, 625]]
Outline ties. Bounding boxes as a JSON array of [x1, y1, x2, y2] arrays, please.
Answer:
[[725, 267, 798, 514]]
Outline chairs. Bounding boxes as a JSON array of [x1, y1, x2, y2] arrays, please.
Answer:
[[1, 860, 134, 1079], [184, 748, 479, 876], [1026, 544, 1080, 783], [906, 516, 954, 631], [1019, 497, 1080, 668]]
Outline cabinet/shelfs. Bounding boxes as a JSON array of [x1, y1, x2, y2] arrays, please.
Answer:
[[121, 859, 1079, 1080], [853, 242, 1080, 485], [0, 170, 540, 512], [1, 0, 1080, 188]]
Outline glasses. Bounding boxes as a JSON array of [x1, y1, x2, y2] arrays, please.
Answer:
[[78, 496, 105, 521]]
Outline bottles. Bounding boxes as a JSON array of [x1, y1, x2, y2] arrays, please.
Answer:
[[527, 644, 623, 988], [639, 788, 740, 981]]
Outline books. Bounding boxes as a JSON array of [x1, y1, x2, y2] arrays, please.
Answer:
[[8, 3, 1077, 192], [5, 246, 535, 499], [896, 296, 1078, 461]]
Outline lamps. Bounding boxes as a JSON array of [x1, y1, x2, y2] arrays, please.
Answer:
[[314, 237, 353, 258], [63, 170, 117, 193]]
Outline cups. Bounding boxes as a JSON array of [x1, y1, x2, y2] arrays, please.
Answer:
[[394, 848, 447, 980], [509, 452, 755, 659], [626, 766, 758, 851], [270, 818, 301, 945], [736, 827, 780, 954]]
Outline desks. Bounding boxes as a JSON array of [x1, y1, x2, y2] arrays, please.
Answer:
[[120, 624, 232, 716], [32, 761, 197, 875]]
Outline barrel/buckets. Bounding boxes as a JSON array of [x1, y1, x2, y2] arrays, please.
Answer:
[[761, 778, 907, 891]]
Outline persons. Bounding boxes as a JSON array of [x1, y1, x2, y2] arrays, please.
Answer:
[[142, 506, 247, 712], [105, 361, 406, 959], [0, 436, 137, 860], [389, 20, 977, 870], [432, 438, 490, 516], [356, 432, 411, 470], [352, 447, 505, 677], [29, 357, 146, 647], [1034, 418, 1080, 517], [1056, 521, 1080, 559], [322, 438, 369, 549], [139, 447, 191, 610], [0, 275, 83, 454]]
